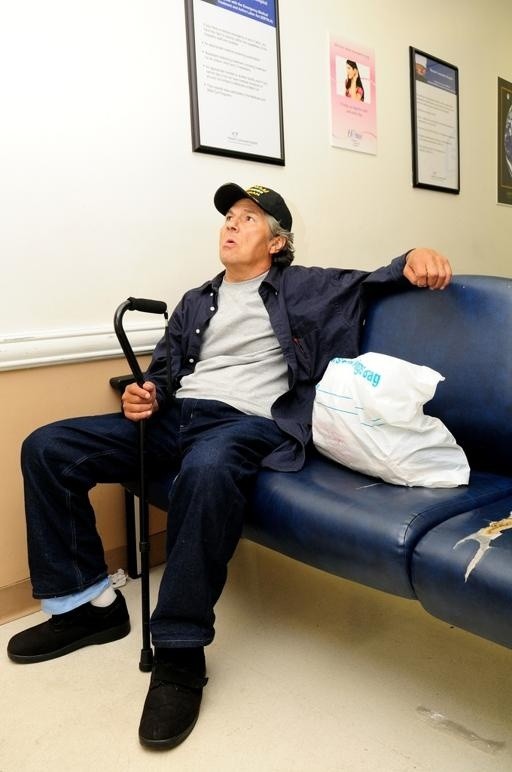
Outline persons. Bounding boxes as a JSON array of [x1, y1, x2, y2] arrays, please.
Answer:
[[10, 179, 453, 748], [343, 60, 365, 103]]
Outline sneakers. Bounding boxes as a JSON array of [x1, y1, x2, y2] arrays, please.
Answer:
[[138, 645, 208, 747], [8, 589, 129, 664]]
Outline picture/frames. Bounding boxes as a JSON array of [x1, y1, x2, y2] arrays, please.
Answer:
[[408, 46, 460, 195], [185, 1, 285, 168]]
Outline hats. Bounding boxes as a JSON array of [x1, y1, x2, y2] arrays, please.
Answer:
[[214, 180, 292, 230]]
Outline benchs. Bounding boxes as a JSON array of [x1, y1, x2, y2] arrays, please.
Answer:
[[107, 275, 510, 651]]
